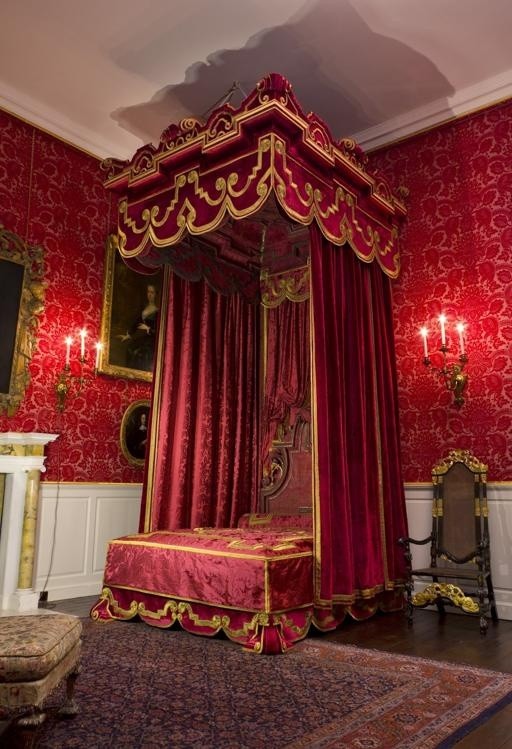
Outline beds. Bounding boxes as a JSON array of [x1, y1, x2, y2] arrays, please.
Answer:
[[90, 72, 411, 655]]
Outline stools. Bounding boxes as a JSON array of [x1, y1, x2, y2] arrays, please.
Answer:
[[0, 615, 82, 749]]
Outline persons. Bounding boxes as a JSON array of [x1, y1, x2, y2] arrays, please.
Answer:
[[115, 284, 160, 371], [130, 413, 148, 458]]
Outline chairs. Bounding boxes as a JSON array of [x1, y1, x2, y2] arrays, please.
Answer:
[[397, 449, 499, 639]]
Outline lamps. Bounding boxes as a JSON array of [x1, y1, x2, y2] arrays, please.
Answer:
[[418, 312, 468, 407], [54, 326, 102, 412]]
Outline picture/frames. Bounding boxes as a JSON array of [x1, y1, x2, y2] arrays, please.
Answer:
[[96, 234, 161, 382], [0, 224, 49, 418], [120, 399, 151, 469]]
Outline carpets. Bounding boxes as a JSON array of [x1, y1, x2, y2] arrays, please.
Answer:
[[1, 617, 511, 749]]
